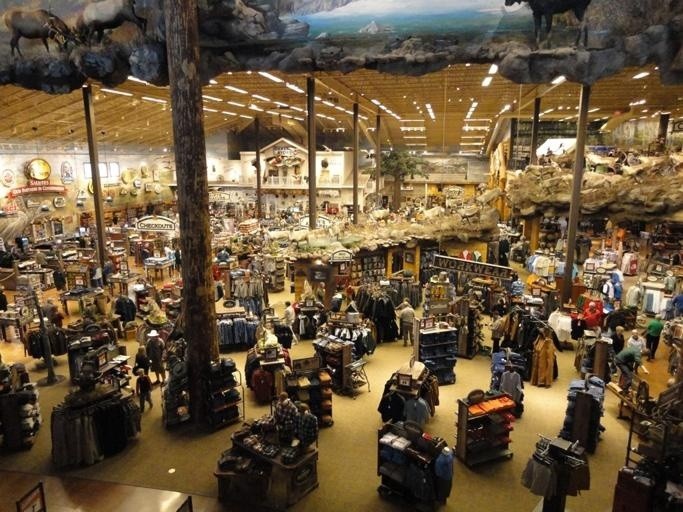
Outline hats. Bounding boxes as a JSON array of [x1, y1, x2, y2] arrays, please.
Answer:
[[147, 330, 160, 337]]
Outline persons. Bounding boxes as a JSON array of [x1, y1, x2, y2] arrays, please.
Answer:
[[293, 402, 320, 450], [626, 283, 641, 315], [93, 259, 115, 290], [484, 311, 503, 353], [608, 314, 664, 395], [316, 281, 326, 302], [603, 279, 622, 302], [279, 301, 299, 345], [510, 277, 525, 297], [394, 297, 415, 346], [45, 297, 64, 328], [53, 265, 67, 292], [435, 447, 453, 504], [134, 330, 165, 415], [672, 288, 683, 317], [274, 392, 298, 429], [164, 244, 182, 272], [583, 301, 601, 326]]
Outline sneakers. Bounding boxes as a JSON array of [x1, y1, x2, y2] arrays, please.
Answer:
[[161, 382, 166, 387], [154, 381, 160, 384]]
